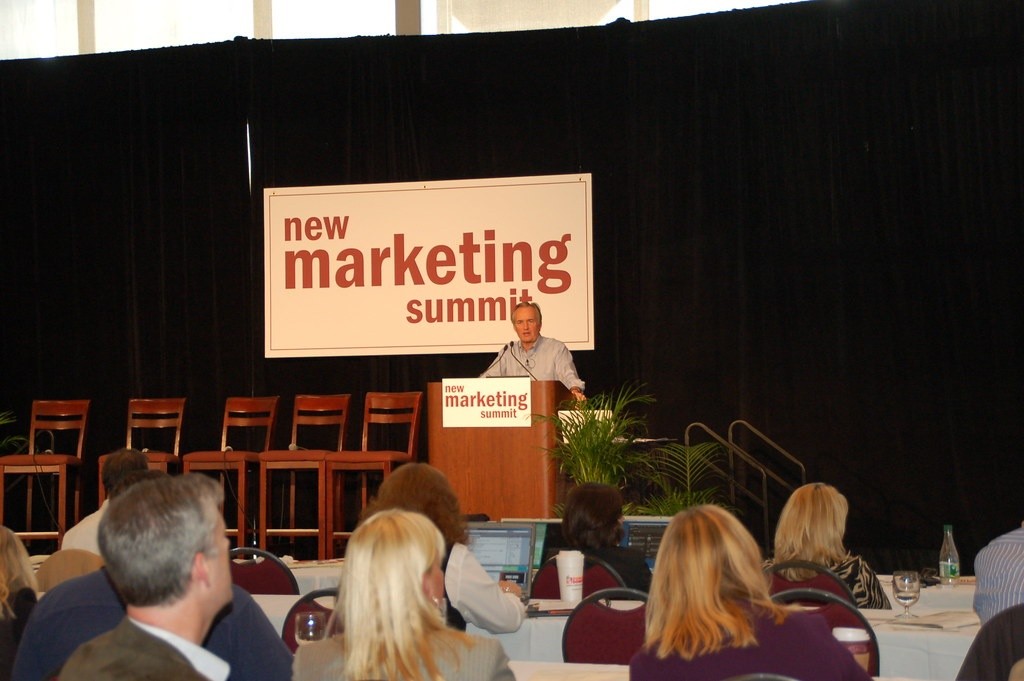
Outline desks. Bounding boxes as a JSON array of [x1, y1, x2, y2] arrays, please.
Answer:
[[249, 558, 981, 681]]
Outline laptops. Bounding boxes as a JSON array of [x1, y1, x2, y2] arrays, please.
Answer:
[[460, 523, 535, 606], [615, 515, 676, 573]]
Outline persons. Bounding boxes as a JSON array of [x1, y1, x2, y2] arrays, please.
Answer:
[[58, 473, 234, 681], [773, 482, 892, 610], [10, 470, 293, 681], [972, 521, 1024, 624], [291, 509, 516, 681], [630, 504, 875, 681], [0, 524, 36, 681], [479, 302, 587, 410], [546, 483, 652, 593], [356, 463, 524, 634], [61, 448, 149, 556]]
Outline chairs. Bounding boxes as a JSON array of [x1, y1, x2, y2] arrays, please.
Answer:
[[281, 585, 345, 655], [769, 589, 882, 681], [226, 548, 302, 596], [563, 585, 649, 667], [531, 551, 626, 601], [0, 391, 425, 560], [765, 560, 860, 608]]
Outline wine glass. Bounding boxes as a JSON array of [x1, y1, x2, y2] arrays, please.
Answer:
[[893, 570, 920, 619]]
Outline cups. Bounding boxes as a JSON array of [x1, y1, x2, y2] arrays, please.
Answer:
[[294, 611, 327, 647]]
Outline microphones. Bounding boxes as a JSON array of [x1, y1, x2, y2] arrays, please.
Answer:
[[478, 345, 508, 378], [510, 341, 538, 382]]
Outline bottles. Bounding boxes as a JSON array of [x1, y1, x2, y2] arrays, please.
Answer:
[[555, 550, 584, 605], [939, 525, 960, 586]]
